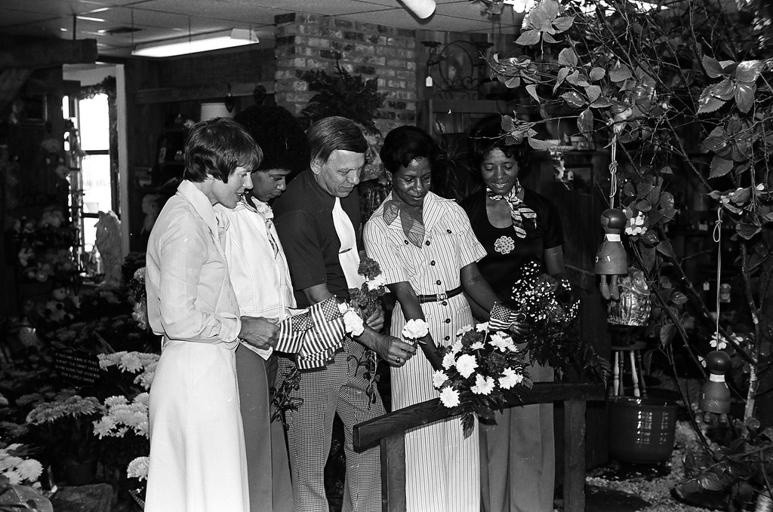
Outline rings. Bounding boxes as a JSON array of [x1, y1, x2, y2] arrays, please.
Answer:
[[396, 357, 400, 363]]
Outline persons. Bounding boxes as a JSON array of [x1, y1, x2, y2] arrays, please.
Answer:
[[212, 133, 310, 510], [460, 140, 558, 512], [269, 116, 416, 511], [365, 126, 506, 512], [143, 118, 283, 512]]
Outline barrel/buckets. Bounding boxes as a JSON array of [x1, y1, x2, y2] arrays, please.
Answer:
[[613, 396, 678, 466]]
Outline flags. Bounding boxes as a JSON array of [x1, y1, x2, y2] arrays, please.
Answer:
[[274, 296, 346, 369], [487, 305, 519, 329]]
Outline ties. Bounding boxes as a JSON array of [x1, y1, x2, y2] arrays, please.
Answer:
[[332, 197, 367, 293]]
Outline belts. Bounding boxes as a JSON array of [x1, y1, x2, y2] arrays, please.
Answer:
[[417, 284, 463, 303]]
